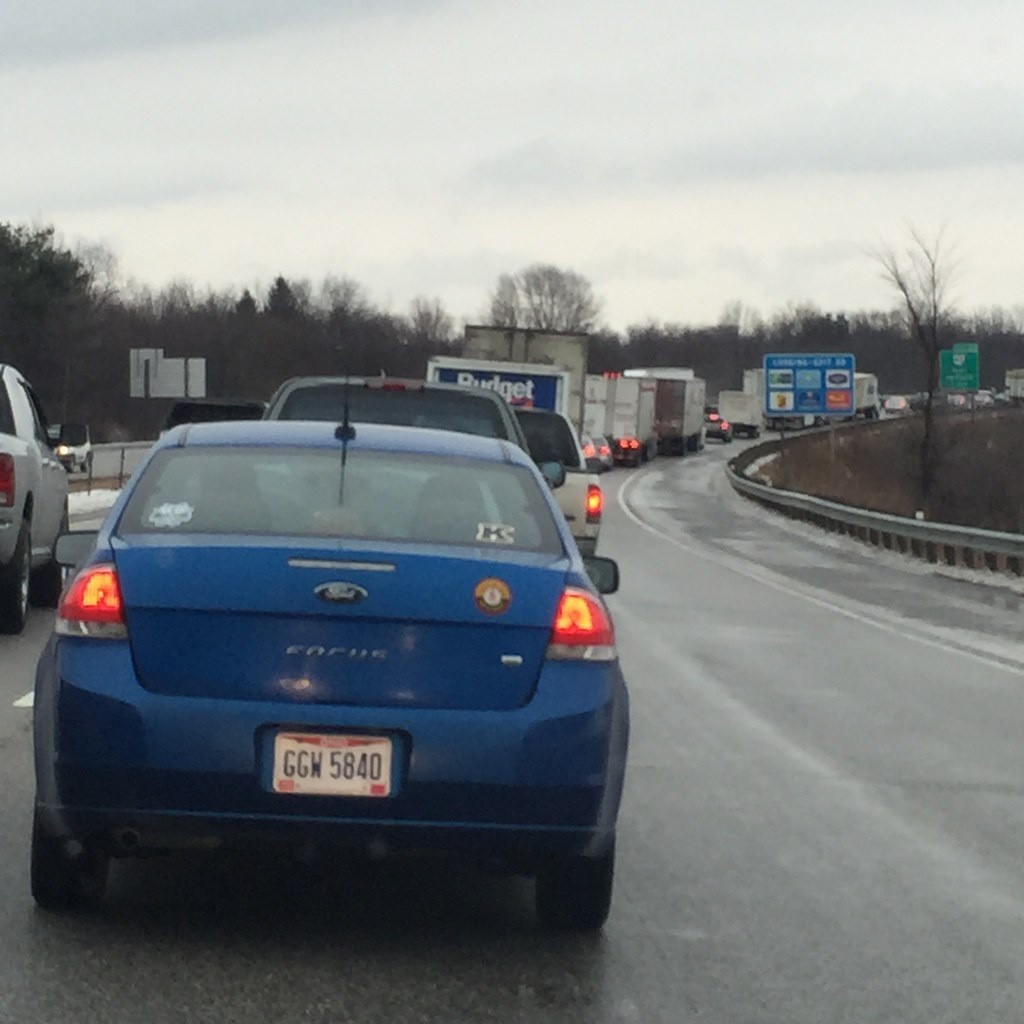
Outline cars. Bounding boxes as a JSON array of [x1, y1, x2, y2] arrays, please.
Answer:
[[948, 391, 1013, 408], [703, 404, 724, 438], [884, 396, 909, 413], [29, 421, 631, 930], [264, 376, 532, 458], [45, 422, 94, 472]]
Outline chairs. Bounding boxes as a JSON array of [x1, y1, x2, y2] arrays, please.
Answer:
[[187, 455, 273, 537], [410, 472, 495, 548]]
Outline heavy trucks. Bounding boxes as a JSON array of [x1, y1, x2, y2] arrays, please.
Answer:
[[743, 369, 884, 430]]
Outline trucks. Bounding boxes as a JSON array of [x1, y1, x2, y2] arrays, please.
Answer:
[[427, 356, 569, 413], [718, 390, 763, 438], [583, 374, 659, 467], [633, 376, 706, 456], [463, 325, 588, 440]]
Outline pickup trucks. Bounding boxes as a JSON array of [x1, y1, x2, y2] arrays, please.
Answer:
[[0, 363, 69, 635], [512, 408, 605, 556]]
